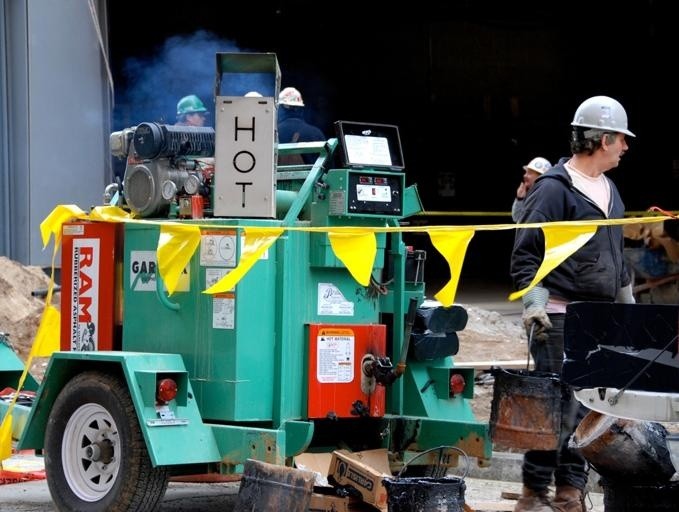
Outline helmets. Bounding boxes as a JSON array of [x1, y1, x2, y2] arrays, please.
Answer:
[[571, 96, 635, 138], [278, 88, 305, 106], [177, 97, 207, 114], [523, 157, 552, 175]]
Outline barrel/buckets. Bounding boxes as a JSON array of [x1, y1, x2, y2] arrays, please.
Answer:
[[232, 458, 318, 512], [382, 446, 469, 512], [567, 410, 675, 484], [488, 321, 563, 452]]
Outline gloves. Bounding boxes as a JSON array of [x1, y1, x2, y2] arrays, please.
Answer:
[[521, 287, 553, 340], [616, 284, 635, 303]]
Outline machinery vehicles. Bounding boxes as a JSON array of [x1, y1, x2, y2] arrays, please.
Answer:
[[0, 51, 492, 512]]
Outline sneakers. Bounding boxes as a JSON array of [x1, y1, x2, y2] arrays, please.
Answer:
[[514, 484, 562, 512], [550, 483, 594, 512]]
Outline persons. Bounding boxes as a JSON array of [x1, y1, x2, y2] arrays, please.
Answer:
[[274, 86, 328, 165], [174, 93, 209, 128], [510, 157, 554, 224], [509, 95, 636, 511]]
